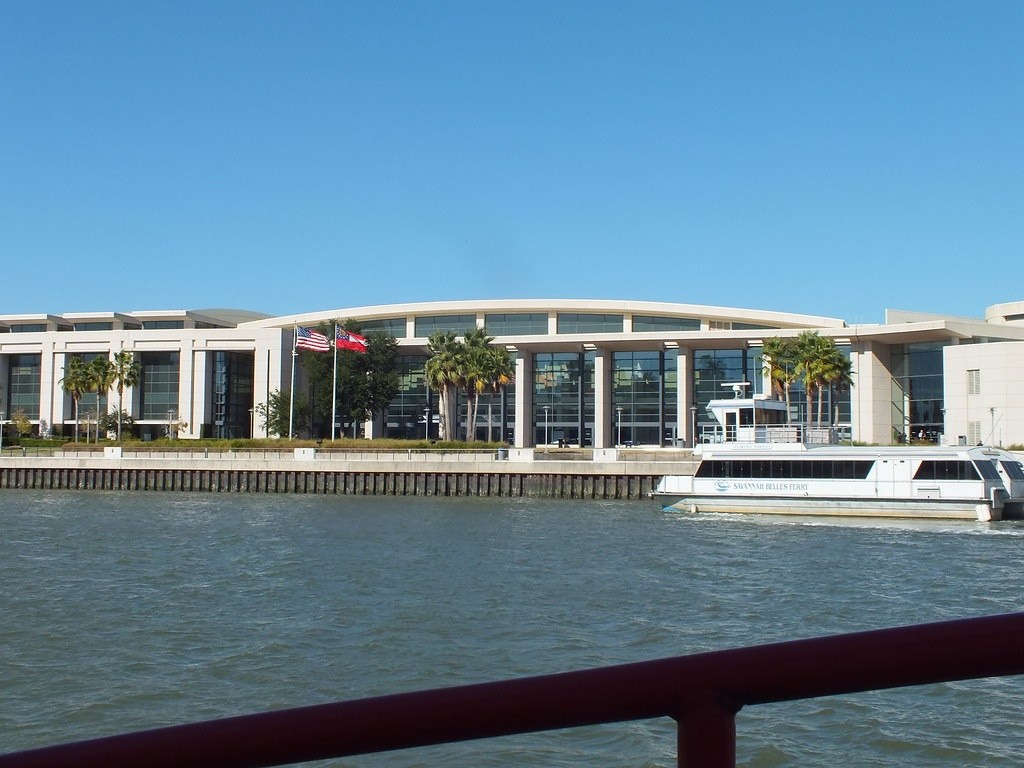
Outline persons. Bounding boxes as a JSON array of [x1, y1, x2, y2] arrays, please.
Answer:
[[918, 430, 926, 441], [977, 441, 983, 446]]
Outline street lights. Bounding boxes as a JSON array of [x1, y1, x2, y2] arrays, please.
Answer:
[[615, 406, 624, 448], [689, 406, 698, 449], [542, 406, 551, 455], [424, 406, 431, 442], [248, 408, 254, 439]]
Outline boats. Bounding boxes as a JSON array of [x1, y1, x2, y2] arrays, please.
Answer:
[[646, 441, 1024, 523]]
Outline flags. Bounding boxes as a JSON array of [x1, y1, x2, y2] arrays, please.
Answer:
[[336, 325, 367, 352], [295, 324, 330, 352]]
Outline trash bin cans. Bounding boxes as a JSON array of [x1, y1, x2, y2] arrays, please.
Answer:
[[677, 438, 684, 448], [958, 435, 966, 445], [498, 448, 505, 460]]
